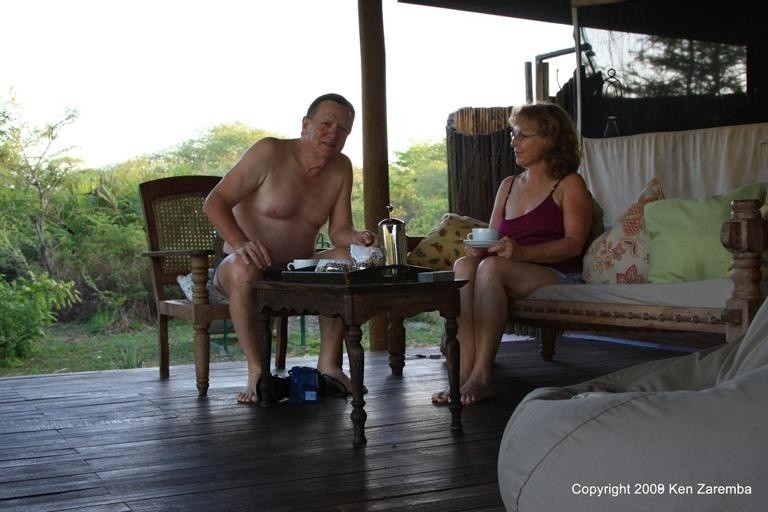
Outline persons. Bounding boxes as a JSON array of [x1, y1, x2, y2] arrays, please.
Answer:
[[201, 92, 368, 406], [430, 100, 594, 405]]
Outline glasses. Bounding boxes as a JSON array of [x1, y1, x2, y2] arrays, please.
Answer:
[[510, 130, 539, 140]]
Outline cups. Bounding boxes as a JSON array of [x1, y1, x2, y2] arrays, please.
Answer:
[[287, 259, 318, 271], [467, 227, 498, 242]]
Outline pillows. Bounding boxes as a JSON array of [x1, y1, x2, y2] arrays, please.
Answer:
[[581, 176, 664, 284], [406, 212, 491, 273], [642, 181, 768, 284]]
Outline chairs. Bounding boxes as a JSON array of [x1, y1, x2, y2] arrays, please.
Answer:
[[498, 298, 768, 511], [139, 175, 288, 397]]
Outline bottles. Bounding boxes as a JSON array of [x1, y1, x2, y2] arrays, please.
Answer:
[[377, 218, 408, 279]]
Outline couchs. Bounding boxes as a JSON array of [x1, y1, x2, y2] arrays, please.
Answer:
[[386, 124, 768, 380]]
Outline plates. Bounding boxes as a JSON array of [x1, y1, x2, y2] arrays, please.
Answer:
[[463, 240, 504, 248]]
